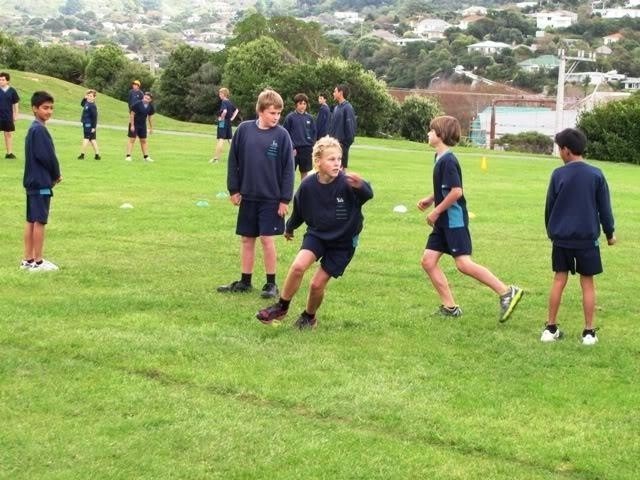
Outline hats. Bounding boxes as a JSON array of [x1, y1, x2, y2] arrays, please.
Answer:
[[132, 80, 141, 86]]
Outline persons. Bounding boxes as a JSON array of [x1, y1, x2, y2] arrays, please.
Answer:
[[283, 83, 357, 180], [21, 91, 62, 272], [257, 135, 373, 331], [127, 80, 144, 115], [417, 116, 524, 324], [78, 90, 102, 160], [540, 128, 616, 345], [125, 92, 155, 162], [209, 87, 239, 164], [0, 73, 21, 158], [217, 88, 295, 300]]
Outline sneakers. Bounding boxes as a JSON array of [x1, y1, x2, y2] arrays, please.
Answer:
[[499, 285, 524, 323], [19, 258, 59, 272], [540, 328, 565, 342], [255, 303, 289, 325], [76, 153, 102, 161], [292, 314, 318, 329], [124, 156, 132, 161], [217, 280, 253, 294], [581, 330, 600, 345], [5, 153, 18, 160], [143, 156, 154, 163], [208, 157, 220, 164], [427, 303, 464, 319], [260, 282, 280, 298]]
